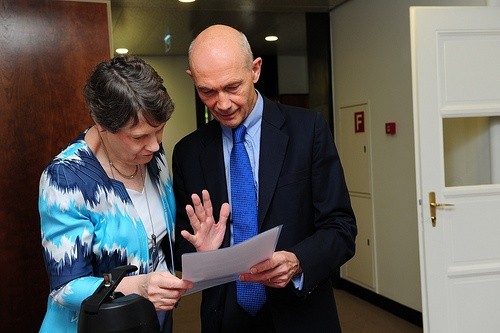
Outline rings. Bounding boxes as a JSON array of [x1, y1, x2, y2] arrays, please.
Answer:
[[268, 278, 271, 283]]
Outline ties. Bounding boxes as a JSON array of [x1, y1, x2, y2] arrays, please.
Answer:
[[222, 125, 267, 317]]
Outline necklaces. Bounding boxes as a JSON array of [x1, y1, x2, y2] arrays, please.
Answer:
[[97, 132, 141, 180]]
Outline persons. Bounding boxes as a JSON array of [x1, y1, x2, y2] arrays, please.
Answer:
[[172, 24, 358, 333], [37, 54, 194, 333]]
